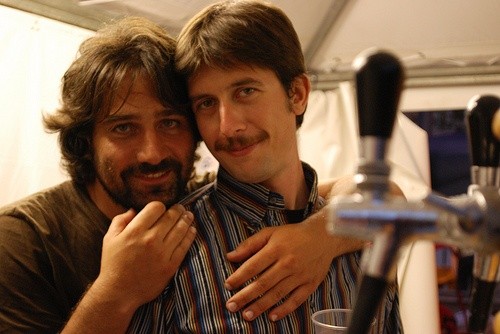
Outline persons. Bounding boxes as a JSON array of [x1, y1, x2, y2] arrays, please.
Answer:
[[127, 2, 404, 334], [1, 17, 375, 334]]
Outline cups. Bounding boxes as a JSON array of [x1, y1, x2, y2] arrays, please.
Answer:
[[308, 307, 376, 334]]
